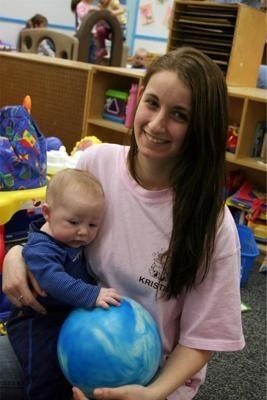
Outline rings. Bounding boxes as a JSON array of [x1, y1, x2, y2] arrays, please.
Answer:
[[15, 294, 24, 301]]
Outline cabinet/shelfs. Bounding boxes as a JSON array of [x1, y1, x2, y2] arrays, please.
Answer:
[[81, 65, 267, 269], [167, 0, 266, 88]]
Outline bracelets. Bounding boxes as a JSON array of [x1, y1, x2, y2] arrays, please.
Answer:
[[11, 241, 24, 247]]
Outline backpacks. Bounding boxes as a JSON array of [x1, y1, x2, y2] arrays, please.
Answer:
[[0, 102, 51, 193]]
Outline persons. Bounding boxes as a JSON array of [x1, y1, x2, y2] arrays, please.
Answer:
[[6, 168, 123, 399], [95, 0, 127, 59], [75, 0, 98, 63], [26, 13, 55, 57], [131, 47, 149, 69], [0, 45, 248, 400]]
